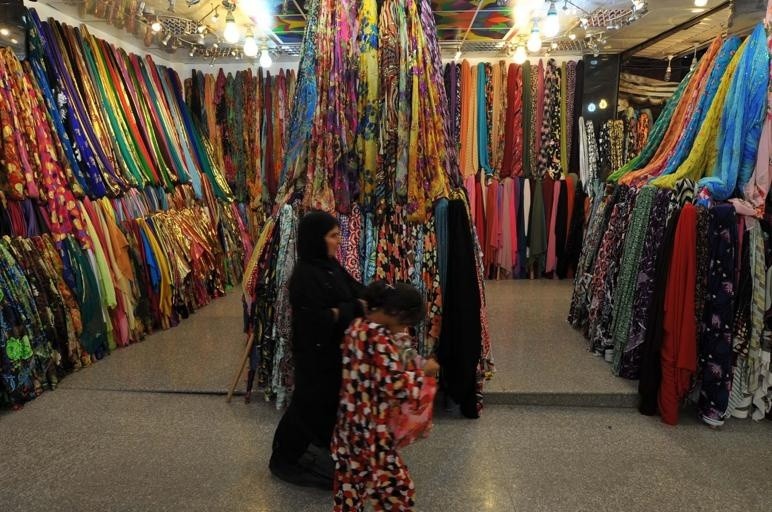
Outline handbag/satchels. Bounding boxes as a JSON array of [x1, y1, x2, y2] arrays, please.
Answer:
[[390, 376, 437, 449]]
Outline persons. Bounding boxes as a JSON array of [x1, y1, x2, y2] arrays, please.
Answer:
[[331, 282, 440, 512], [268, 212, 391, 490]]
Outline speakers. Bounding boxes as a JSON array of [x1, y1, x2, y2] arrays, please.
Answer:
[[575, 53, 621, 123]]
